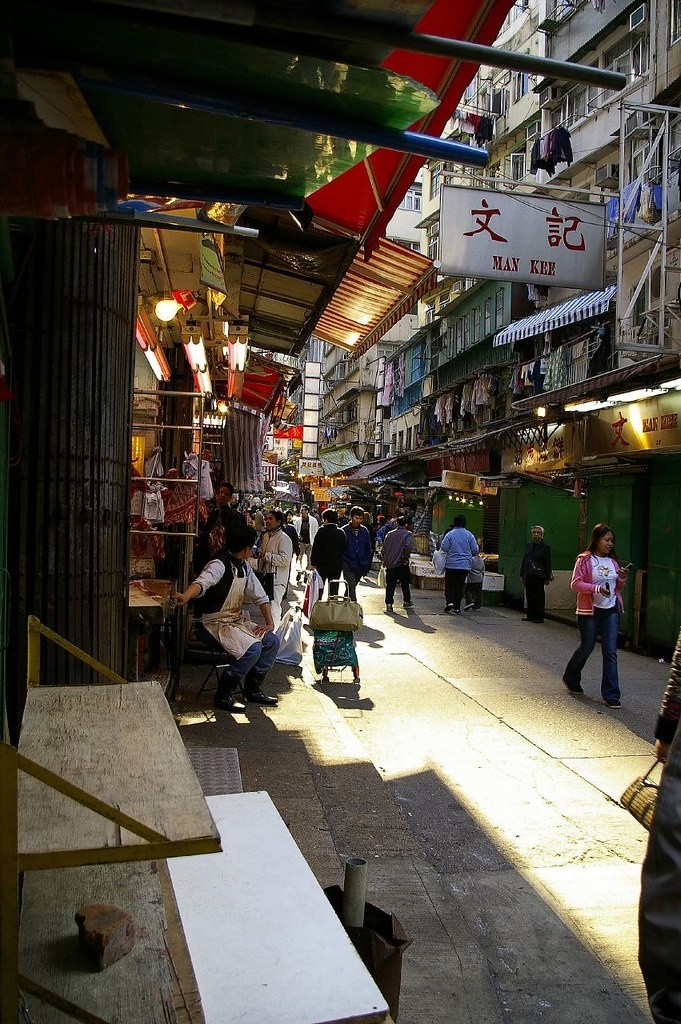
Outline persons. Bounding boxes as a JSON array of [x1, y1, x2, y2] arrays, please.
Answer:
[[198, 481, 376, 600], [377, 506, 416, 612], [441, 514, 485, 613], [561, 522, 632, 710], [310, 508, 347, 602], [171, 524, 279, 713], [341, 506, 374, 601], [519, 524, 552, 625], [654, 632, 681, 765]]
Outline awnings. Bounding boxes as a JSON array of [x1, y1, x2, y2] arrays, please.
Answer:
[[334, 457, 407, 486], [510, 352, 680, 411], [306, 0, 516, 268], [311, 228, 439, 363], [238, 355, 304, 428], [493, 283, 618, 349]]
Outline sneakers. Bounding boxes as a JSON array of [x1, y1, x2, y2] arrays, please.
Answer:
[[386, 604, 393, 611], [402, 602, 413, 609]]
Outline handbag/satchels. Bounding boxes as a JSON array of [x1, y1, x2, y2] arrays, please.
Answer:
[[301, 569, 323, 620], [254, 572, 275, 602], [533, 562, 546, 578], [268, 605, 303, 666], [620, 761, 664, 832], [432, 548, 446, 576], [377, 565, 387, 588], [310, 595, 363, 633]]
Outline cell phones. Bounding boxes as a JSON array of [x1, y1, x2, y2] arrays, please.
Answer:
[[626, 563, 634, 569]]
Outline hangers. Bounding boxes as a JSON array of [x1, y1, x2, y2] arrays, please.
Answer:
[[472, 373, 497, 379], [438, 382, 470, 398]]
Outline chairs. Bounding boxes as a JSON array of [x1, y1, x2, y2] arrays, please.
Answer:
[[176, 561, 237, 706]]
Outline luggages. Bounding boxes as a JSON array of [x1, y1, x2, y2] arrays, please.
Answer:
[[312, 579, 358, 674]]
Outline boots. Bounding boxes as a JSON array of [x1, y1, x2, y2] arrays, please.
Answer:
[[242, 670, 279, 705], [212, 669, 246, 712]]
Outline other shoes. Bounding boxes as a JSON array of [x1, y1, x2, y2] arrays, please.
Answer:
[[530, 620, 544, 623], [444, 603, 454, 611], [463, 602, 475, 611], [453, 609, 461, 612], [561, 677, 584, 695], [520, 617, 531, 621], [471, 608, 480, 612], [604, 699, 622, 709]]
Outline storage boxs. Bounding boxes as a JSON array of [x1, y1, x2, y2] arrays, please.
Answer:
[[404, 553, 504, 605]]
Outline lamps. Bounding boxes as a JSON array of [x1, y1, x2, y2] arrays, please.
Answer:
[[446, 492, 486, 506], [537, 373, 681, 418], [136, 292, 247, 390]]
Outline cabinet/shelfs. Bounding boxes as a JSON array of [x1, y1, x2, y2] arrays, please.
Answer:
[[0, 614, 225, 1023]]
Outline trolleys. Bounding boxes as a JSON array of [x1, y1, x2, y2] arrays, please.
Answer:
[[309, 579, 361, 685]]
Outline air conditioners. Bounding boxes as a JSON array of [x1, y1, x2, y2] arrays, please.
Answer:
[[340, 354, 347, 362], [452, 280, 466, 293], [594, 164, 622, 190]]
